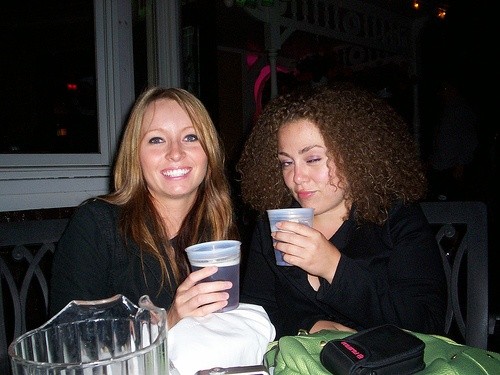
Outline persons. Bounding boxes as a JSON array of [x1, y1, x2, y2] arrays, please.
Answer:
[[48, 86, 233, 330], [236, 90, 449, 337]]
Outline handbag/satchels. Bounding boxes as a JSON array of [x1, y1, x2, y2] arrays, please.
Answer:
[[263, 329, 500, 375], [320, 327, 425, 375]]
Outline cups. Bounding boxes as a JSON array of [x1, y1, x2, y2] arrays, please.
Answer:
[[185, 240, 242, 313], [7, 294, 169, 375], [266, 208, 313, 266]]
